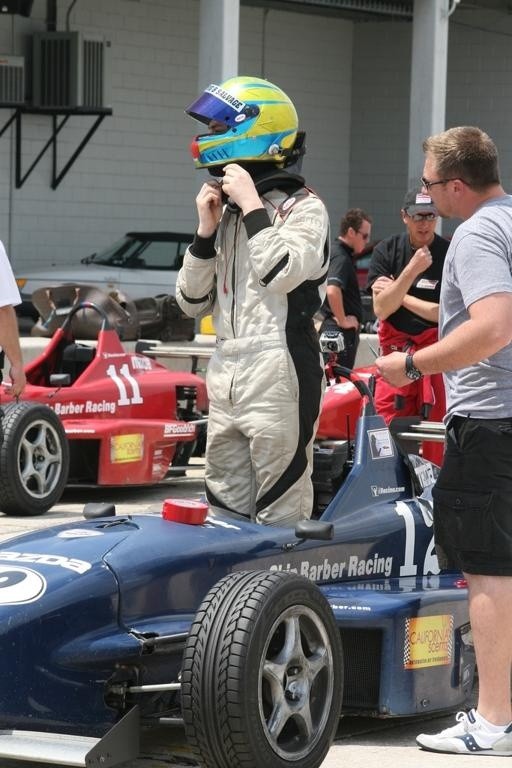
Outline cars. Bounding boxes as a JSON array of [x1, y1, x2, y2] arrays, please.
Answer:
[[354, 242, 387, 309], [12, 231, 208, 340]]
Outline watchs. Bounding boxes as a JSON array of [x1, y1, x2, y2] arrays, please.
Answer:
[[405, 354, 425, 380]]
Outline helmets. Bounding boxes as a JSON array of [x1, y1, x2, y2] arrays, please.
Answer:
[[184, 75, 306, 171]]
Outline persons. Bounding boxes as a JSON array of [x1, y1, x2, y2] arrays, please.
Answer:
[[374, 127, 512, 756], [367, 188, 450, 467], [0, 237, 26, 399], [175, 73, 331, 529], [317, 210, 371, 370]]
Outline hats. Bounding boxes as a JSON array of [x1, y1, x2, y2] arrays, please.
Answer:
[[401, 185, 440, 216]]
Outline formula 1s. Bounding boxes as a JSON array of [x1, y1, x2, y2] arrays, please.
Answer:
[[0, 347, 478, 766], [0, 302, 215, 511], [306, 337, 453, 516]]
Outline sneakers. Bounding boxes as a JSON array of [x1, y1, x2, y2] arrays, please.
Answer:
[[415, 708, 512, 756]]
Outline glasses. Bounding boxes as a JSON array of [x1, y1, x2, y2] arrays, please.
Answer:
[[407, 214, 438, 222], [420, 176, 472, 191], [355, 230, 369, 241]]
